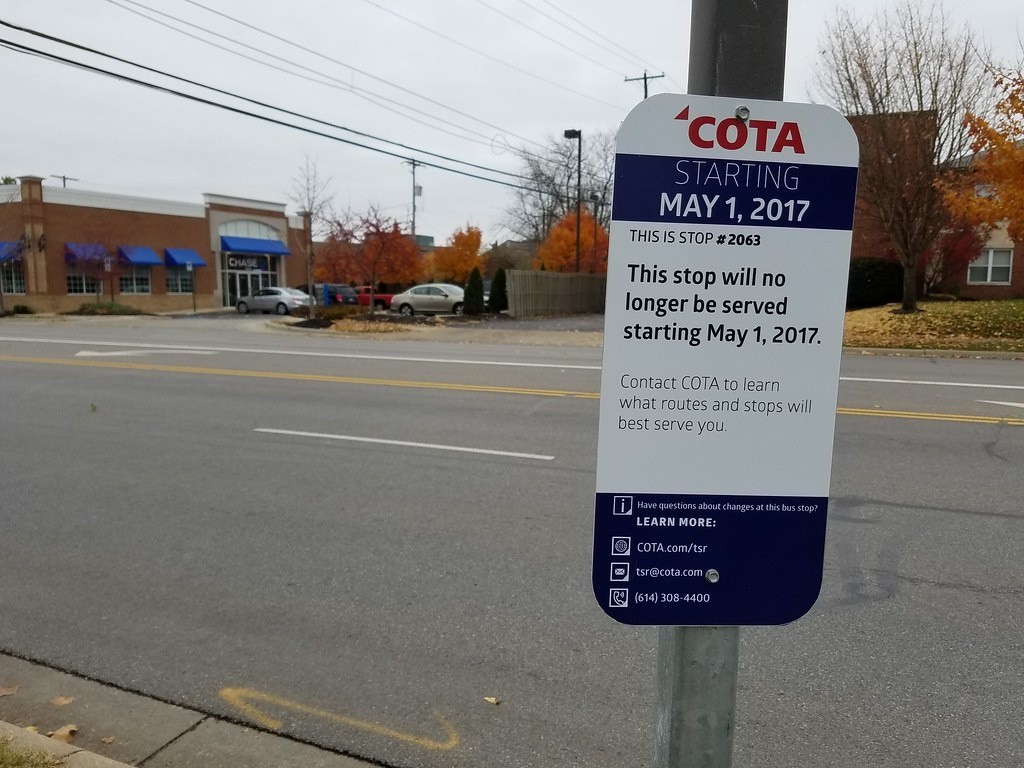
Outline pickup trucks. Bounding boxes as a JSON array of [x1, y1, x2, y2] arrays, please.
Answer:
[[353, 285, 396, 311]]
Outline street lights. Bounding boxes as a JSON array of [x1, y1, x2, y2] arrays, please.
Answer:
[[564, 128, 582, 272]]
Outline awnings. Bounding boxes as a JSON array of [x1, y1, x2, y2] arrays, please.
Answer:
[[166, 248, 206, 267], [0, 242, 22, 262], [222, 235, 291, 256], [118, 245, 164, 267], [68, 243, 109, 264]]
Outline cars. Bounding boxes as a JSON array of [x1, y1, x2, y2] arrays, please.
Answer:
[[236, 286, 317, 315], [390, 282, 490, 317]]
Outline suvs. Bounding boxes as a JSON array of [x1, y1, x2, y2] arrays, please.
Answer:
[[296, 283, 359, 307]]
